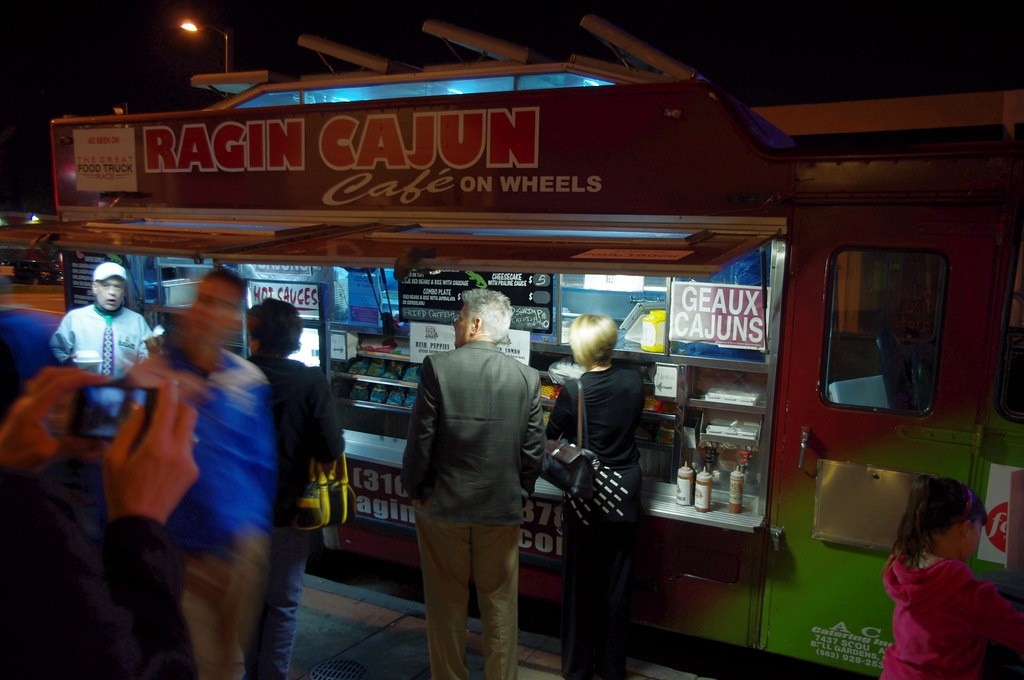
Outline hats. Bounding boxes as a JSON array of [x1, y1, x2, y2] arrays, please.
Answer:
[[92, 262, 129, 282]]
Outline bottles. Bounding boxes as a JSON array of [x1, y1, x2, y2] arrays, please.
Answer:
[[694, 465, 712, 512], [675, 461, 694, 506], [640, 310, 669, 352], [728, 465, 745, 514]]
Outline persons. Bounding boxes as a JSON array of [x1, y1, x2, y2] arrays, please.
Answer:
[[401, 289, 545, 680], [545, 315, 646, 680], [0, 262, 344, 680], [879, 475, 1024, 680]]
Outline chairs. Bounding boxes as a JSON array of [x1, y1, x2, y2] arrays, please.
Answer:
[[876, 332, 909, 411]]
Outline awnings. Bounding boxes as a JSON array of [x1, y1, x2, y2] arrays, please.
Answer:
[[56, 215, 780, 278]]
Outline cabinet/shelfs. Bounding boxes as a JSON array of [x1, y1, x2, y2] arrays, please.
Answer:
[[682, 357, 777, 500], [133, 255, 680, 486]]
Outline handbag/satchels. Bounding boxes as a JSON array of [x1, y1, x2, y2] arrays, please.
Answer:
[[564, 378, 629, 526], [542, 379, 596, 500], [294, 451, 356, 531]]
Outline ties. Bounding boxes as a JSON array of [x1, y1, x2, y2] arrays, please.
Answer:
[[101, 316, 114, 377]]
